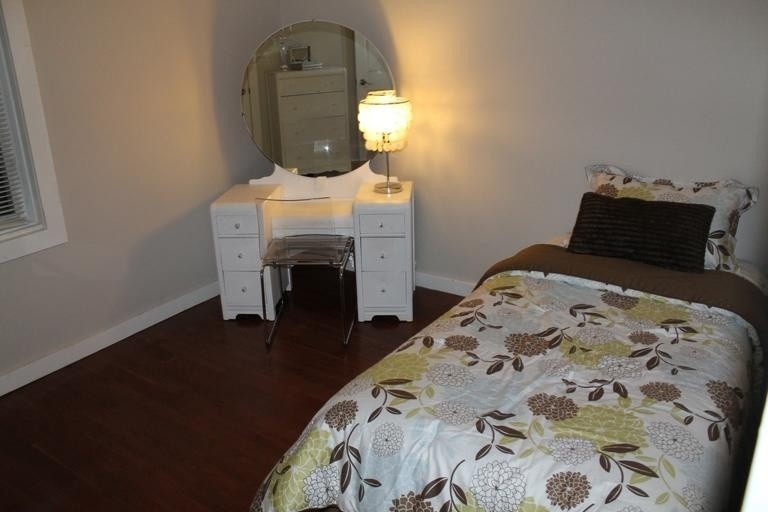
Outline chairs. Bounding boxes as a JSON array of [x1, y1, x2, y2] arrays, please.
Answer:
[[253, 197, 355, 345]]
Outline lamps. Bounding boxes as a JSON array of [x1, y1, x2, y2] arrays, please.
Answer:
[[358, 89, 411, 194]]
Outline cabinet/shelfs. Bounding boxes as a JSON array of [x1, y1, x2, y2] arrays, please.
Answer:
[[265, 64, 352, 175]]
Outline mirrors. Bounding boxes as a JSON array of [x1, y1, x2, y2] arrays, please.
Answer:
[[240, 18, 400, 179]]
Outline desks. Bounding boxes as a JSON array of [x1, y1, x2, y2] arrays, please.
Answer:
[[209, 174, 416, 324]]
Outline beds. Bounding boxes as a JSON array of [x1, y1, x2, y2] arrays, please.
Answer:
[[251, 235, 768, 512]]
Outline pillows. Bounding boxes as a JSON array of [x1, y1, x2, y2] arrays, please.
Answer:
[[566, 162, 759, 279]]
